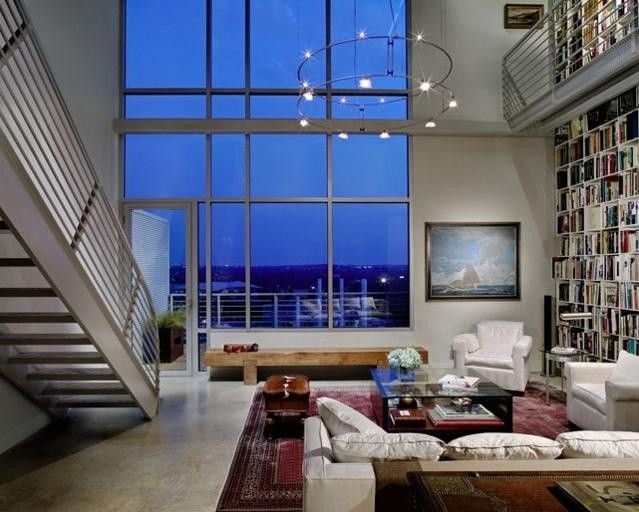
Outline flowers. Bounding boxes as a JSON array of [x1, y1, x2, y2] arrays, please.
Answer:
[[387, 349, 421, 370]]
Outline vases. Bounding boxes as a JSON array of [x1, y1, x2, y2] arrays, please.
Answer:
[[400, 369, 415, 381]]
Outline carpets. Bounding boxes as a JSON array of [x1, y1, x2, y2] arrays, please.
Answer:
[[212, 380, 639, 512]]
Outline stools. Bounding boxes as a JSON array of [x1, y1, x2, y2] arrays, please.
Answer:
[[262, 374, 310, 418]]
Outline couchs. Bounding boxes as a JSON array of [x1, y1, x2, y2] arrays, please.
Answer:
[[451, 319, 533, 392], [566, 349, 639, 432], [302, 417, 639, 512]]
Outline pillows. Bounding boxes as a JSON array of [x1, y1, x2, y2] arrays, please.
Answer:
[[556, 431, 639, 458], [316, 395, 388, 434], [330, 433, 446, 462], [446, 432, 566, 459]]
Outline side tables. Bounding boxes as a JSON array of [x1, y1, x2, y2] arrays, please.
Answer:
[[540, 348, 586, 404]]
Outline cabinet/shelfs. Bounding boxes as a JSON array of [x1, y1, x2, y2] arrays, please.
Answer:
[[550, 0, 639, 359]]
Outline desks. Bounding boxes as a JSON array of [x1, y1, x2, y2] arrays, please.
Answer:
[[407, 471, 639, 512], [369, 366, 515, 440]]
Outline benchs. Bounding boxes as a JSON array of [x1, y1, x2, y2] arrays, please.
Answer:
[[206, 347, 428, 385]]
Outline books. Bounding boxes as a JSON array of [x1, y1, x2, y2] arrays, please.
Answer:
[[543, 0, 638, 86], [388, 406, 427, 428], [555, 88, 639, 371], [427, 401, 506, 429]]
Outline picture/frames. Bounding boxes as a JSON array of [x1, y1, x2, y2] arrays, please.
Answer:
[[425, 222, 521, 302], [504, 3, 544, 29]]
